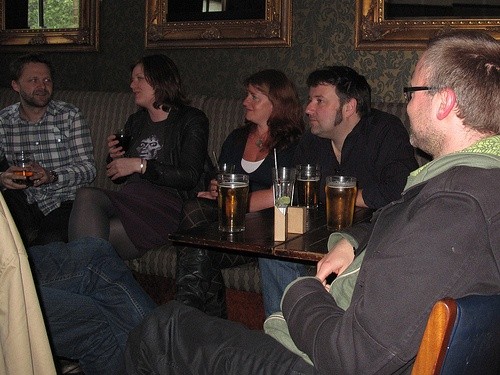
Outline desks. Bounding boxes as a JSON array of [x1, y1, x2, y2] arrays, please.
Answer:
[[166, 203, 377, 284]]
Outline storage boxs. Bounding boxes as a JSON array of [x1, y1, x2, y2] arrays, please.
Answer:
[[273, 205, 307, 242]]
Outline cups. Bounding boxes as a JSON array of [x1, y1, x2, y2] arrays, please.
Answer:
[[324, 176, 357, 232], [296, 162, 321, 209], [214, 162, 236, 180], [271, 167, 296, 208], [12, 151, 35, 179], [216, 173, 249, 234]]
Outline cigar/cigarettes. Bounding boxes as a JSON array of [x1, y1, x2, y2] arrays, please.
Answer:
[[15, 171, 33, 176]]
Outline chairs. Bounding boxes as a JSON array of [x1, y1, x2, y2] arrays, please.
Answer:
[[411, 293, 500, 375]]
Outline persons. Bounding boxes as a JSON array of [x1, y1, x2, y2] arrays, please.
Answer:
[[259, 65, 421, 317], [173, 70, 305, 319], [0, 54, 97, 248], [68, 54, 218, 261], [128, 30, 500, 375], [25, 192, 157, 375]]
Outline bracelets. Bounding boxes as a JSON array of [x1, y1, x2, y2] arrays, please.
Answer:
[[140, 158, 143, 174]]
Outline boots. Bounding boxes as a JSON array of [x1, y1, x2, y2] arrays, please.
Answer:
[[173, 227, 228, 319]]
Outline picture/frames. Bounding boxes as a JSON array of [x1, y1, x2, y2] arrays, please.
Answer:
[[355, 0, 500, 51], [144, 0, 292, 50], [0, 0, 100, 52]]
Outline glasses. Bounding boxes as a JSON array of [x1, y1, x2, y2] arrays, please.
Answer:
[[402, 85, 437, 101]]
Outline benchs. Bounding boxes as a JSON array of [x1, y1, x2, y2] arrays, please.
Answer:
[[0, 86, 434, 330]]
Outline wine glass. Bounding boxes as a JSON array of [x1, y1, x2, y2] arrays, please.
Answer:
[[112, 130, 130, 160]]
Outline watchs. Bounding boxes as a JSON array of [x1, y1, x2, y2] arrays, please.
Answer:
[[49, 170, 58, 183]]
